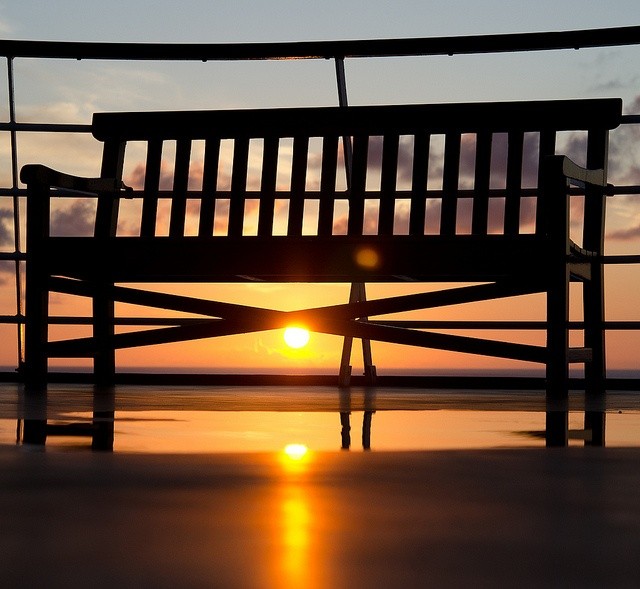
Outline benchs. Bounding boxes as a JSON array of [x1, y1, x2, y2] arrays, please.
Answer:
[[19, 97, 623, 450]]
